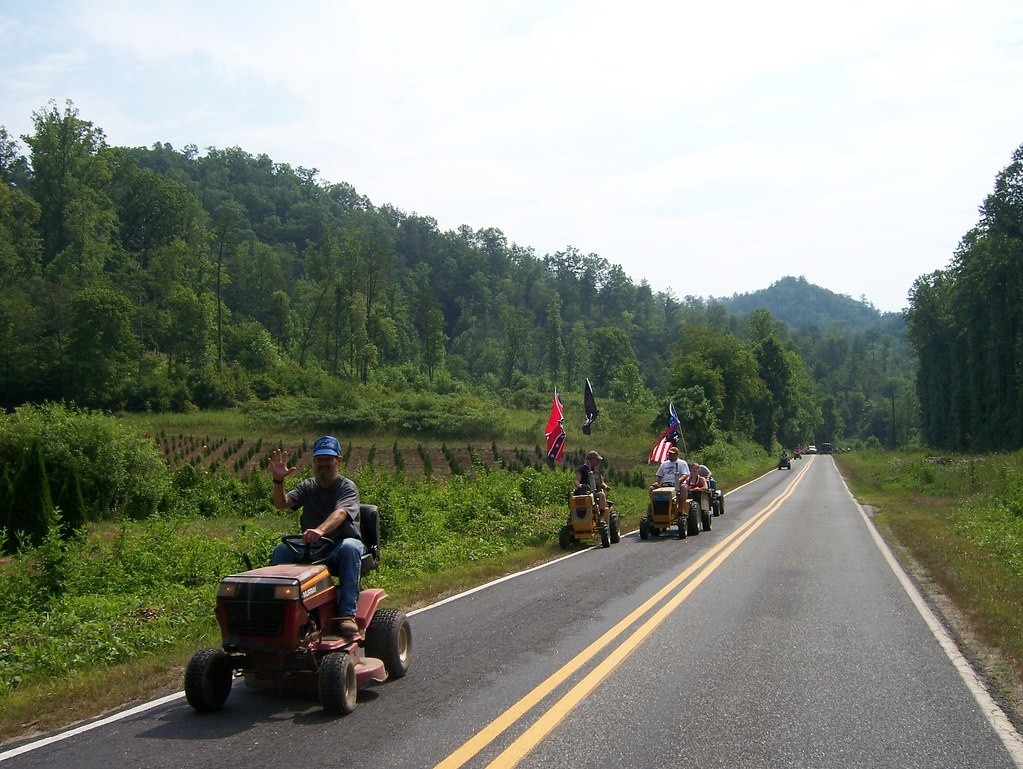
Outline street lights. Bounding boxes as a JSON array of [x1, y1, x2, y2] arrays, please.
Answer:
[[892, 394, 902, 449]]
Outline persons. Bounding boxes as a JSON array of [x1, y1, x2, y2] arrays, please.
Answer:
[[780, 449, 789, 459], [698, 465, 712, 479], [794, 447, 800, 452], [836, 445, 851, 453], [805, 446, 809, 452], [649, 447, 691, 516], [684, 463, 708, 491], [268, 435, 368, 636], [574, 450, 611, 523]]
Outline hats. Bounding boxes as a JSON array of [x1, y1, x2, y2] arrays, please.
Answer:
[[313, 436, 341, 456], [669, 447, 679, 454], [587, 451, 603, 461]]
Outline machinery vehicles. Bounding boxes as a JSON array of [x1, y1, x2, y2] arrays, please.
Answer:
[[559, 460, 621, 549], [185, 505, 413, 718], [777, 454, 790, 470], [640, 462, 701, 539], [704, 474, 724, 516]]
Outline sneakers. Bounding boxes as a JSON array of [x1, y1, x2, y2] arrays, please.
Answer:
[[339, 616, 358, 635]]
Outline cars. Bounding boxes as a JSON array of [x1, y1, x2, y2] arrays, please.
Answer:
[[807, 446, 817, 453], [820, 443, 833, 455]]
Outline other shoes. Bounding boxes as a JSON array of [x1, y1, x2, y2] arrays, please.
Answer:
[[678, 511, 687, 516], [600, 518, 606, 524]]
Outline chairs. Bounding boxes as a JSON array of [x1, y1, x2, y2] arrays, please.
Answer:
[[360, 504, 380, 576]]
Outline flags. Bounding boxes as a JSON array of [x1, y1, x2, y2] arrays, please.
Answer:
[[648, 429, 680, 465], [667, 401, 680, 428], [582, 378, 599, 435], [545, 387, 566, 463]]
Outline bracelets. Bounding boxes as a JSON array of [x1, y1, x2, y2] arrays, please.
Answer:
[[577, 484, 581, 487], [273, 479, 283, 484]]
